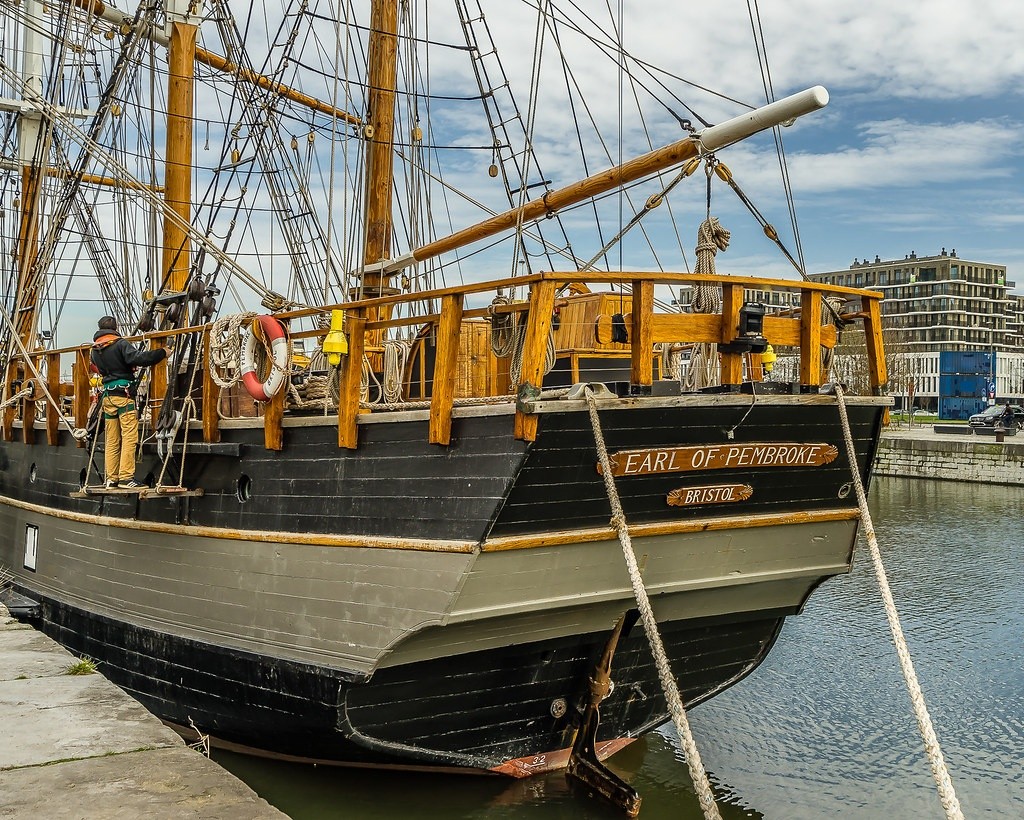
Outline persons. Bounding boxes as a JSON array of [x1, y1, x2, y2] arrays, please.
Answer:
[[91, 316, 173, 489], [1000, 403, 1015, 428]]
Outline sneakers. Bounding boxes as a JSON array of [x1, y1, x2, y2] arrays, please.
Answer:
[[118, 477, 149, 489], [106, 480, 119, 489]]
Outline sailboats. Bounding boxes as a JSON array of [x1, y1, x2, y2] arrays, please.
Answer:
[[0, 1, 907, 783]]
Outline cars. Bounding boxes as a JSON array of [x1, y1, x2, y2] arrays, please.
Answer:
[[913, 409, 934, 416], [894, 410, 900, 415]]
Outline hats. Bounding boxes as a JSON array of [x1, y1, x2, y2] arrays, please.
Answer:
[[98, 316, 117, 329]]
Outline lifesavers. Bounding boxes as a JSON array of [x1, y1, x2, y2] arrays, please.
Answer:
[[236, 314, 287, 400]]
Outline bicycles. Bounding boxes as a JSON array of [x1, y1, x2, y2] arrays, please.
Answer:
[[994, 415, 1022, 434]]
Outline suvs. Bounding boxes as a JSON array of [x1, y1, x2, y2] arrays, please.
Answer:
[[968, 405, 1024, 428]]
